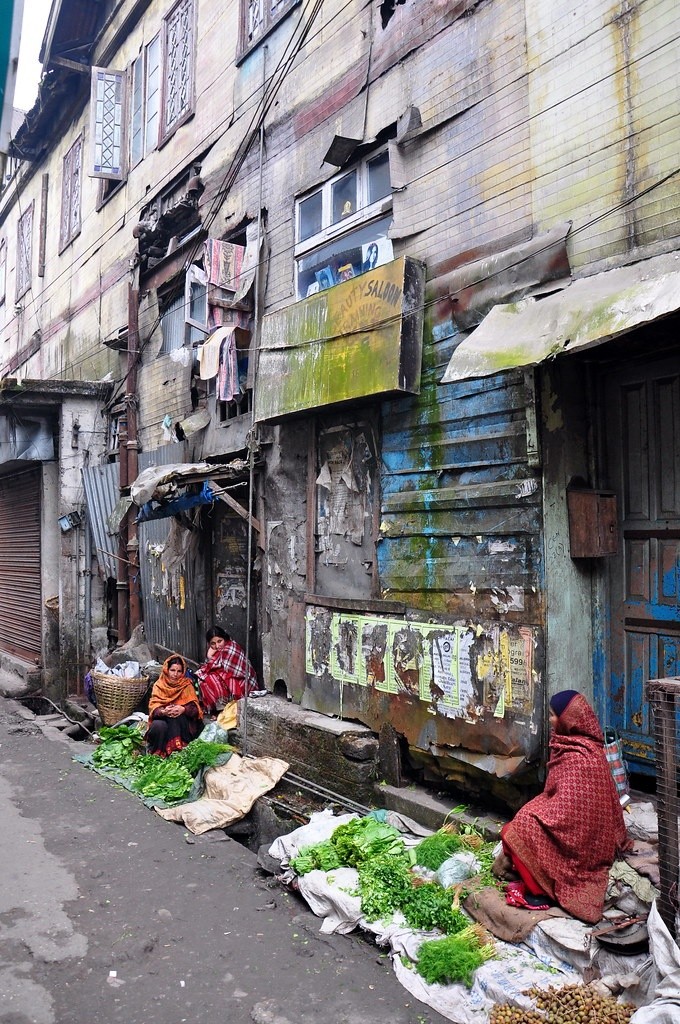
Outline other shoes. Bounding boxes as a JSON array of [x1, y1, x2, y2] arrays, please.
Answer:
[[527, 892, 545, 904]]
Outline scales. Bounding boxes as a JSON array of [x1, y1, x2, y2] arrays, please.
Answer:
[[584, 915, 649, 956]]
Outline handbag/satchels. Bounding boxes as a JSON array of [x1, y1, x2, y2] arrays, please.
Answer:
[[602, 725, 630, 797]]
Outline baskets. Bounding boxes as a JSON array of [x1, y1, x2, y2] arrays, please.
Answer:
[[90, 668, 150, 727]]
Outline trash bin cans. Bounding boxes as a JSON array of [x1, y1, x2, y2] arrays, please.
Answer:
[[91, 672, 151, 727]]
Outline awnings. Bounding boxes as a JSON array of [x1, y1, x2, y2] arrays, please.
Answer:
[[438, 251, 680, 385]]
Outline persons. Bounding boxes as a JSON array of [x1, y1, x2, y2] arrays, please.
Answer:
[[146, 654, 204, 760], [500, 691, 624, 910], [197, 626, 259, 721]]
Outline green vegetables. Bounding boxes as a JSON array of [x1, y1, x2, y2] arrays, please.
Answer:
[[400, 920, 498, 990], [289, 805, 508, 932], [90, 722, 241, 803]]
